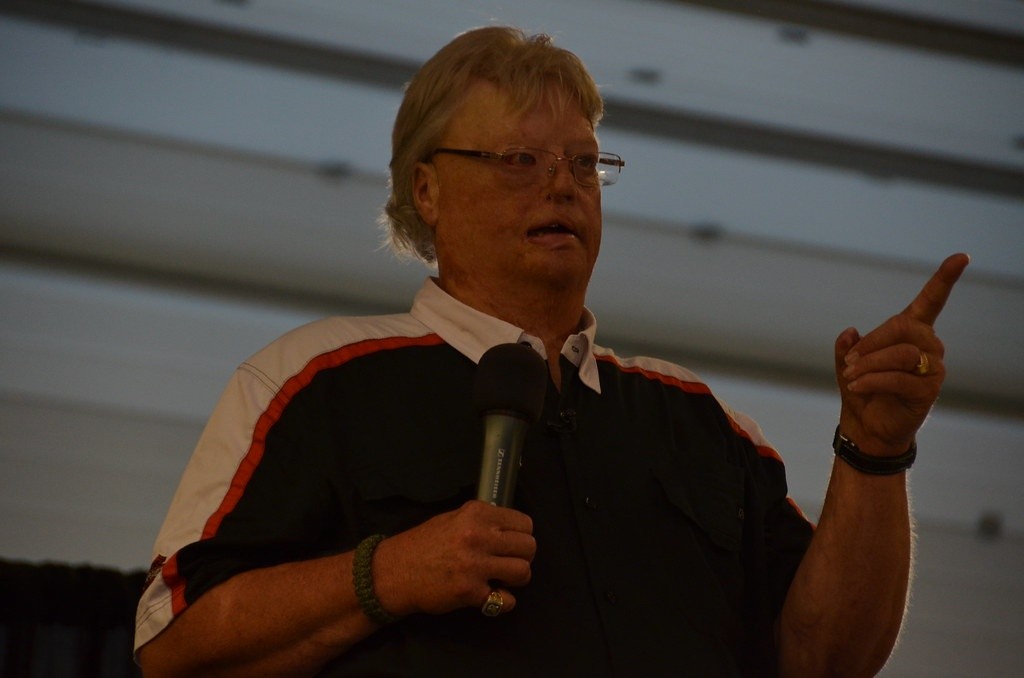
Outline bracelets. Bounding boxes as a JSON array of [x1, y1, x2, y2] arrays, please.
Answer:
[[352, 532, 407, 629], [831, 421, 919, 474]]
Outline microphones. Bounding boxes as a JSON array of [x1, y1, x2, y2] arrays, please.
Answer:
[[476, 342, 547, 508]]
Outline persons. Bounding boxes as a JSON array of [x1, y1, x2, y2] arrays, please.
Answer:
[[129, 26, 974, 677]]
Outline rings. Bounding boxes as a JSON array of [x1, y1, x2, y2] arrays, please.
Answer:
[[915, 347, 933, 377], [478, 588, 505, 616]]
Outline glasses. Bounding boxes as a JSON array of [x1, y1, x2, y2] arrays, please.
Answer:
[[427, 143, 625, 188]]
